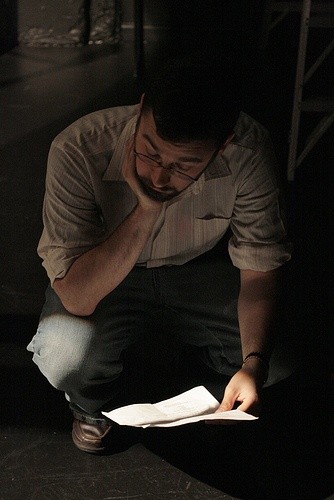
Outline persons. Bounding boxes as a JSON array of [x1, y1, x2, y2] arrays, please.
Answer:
[[25, 54, 304, 455]]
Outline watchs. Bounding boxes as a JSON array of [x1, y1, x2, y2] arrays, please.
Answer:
[[241, 353, 270, 387]]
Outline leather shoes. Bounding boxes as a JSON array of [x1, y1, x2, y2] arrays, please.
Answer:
[[70, 413, 118, 454]]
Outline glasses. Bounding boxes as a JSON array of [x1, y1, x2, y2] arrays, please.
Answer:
[[134, 103, 228, 182]]
